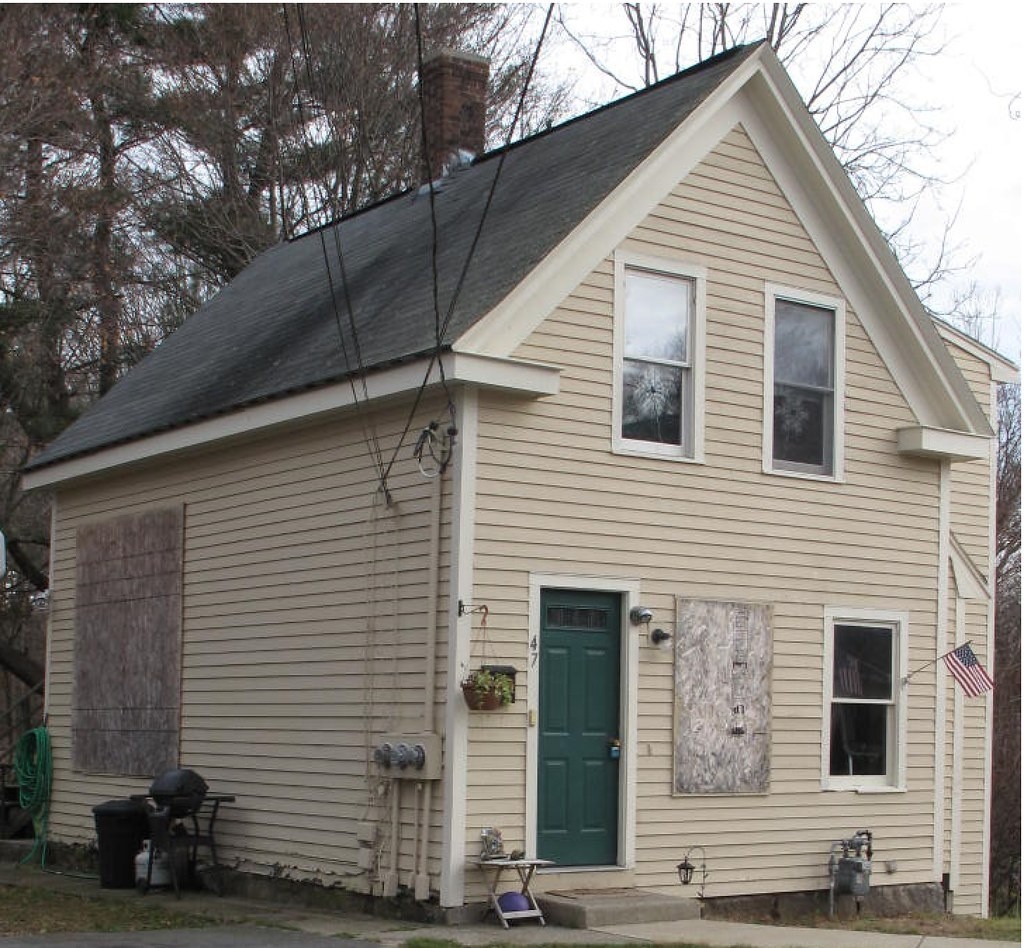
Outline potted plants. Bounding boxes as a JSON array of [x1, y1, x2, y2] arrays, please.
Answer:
[[460, 667, 514, 711]]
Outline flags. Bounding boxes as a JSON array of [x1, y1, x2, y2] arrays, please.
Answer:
[[836, 653, 863, 697], [942, 644, 996, 698]]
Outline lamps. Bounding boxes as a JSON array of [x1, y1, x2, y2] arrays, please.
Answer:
[[650, 629, 676, 653], [630, 607, 652, 627], [677, 846, 709, 903]]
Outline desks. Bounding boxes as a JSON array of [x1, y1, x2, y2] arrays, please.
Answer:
[[466, 858, 556, 930], [129, 794, 237, 900]]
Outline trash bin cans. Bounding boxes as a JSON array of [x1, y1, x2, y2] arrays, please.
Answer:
[[90, 799, 155, 891]]
